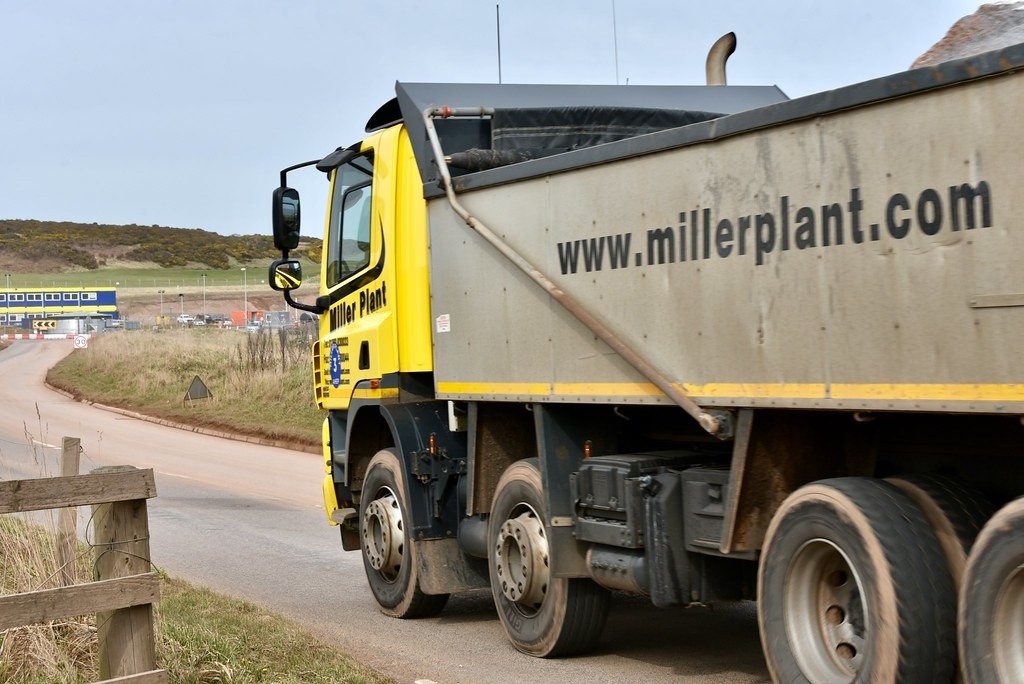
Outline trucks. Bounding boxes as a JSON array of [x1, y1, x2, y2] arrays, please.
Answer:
[[269, 30, 1024, 684]]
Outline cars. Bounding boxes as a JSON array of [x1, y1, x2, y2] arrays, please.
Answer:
[[178, 313, 232, 326]]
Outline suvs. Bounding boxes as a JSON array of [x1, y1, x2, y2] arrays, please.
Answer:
[[247, 320, 262, 333]]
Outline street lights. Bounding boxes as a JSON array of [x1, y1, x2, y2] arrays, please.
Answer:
[[241, 268, 248, 326], [158, 289, 165, 329], [5, 274, 12, 325], [201, 272, 208, 322]]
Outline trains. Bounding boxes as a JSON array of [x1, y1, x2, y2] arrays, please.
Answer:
[[0, 287, 121, 327]]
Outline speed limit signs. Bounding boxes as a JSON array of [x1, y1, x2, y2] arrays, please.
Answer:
[[74, 334, 89, 349]]
